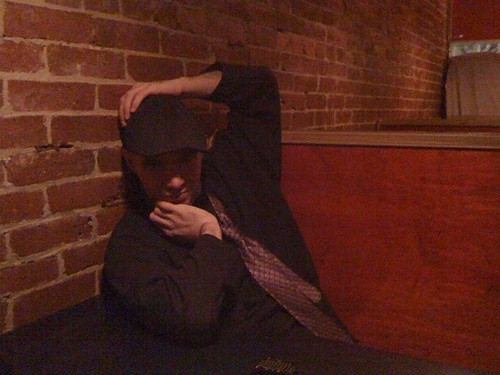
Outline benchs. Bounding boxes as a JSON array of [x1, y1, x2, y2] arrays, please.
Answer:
[[378, 117, 499, 132], [280, 129, 500, 374]]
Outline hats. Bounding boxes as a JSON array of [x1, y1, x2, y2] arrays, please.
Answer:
[[119, 94, 209, 161]]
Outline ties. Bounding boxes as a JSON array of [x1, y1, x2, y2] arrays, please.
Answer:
[[202, 190, 357, 343]]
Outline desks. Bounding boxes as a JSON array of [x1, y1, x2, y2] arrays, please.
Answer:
[[0, 294, 500, 374]]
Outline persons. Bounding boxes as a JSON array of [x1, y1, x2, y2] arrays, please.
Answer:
[[102, 61, 357, 345]]
